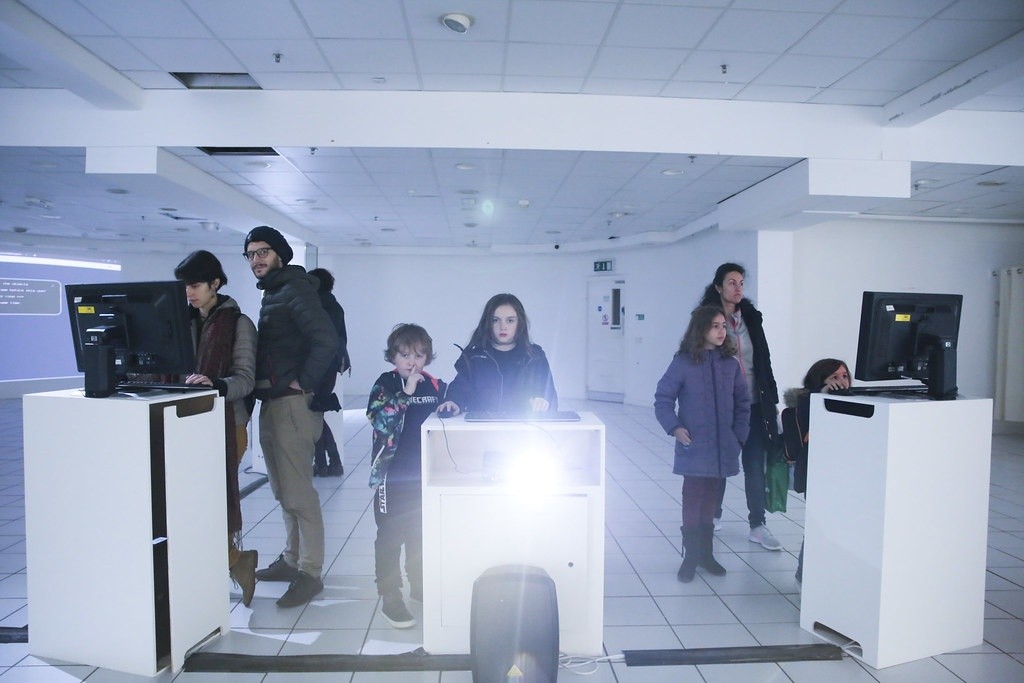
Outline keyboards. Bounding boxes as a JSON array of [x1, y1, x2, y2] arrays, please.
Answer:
[[828, 385, 928, 396], [117, 380, 212, 393], [464, 410, 582, 423]]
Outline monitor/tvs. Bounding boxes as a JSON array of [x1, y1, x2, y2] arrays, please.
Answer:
[[64, 280, 195, 398], [855, 291, 963, 400]]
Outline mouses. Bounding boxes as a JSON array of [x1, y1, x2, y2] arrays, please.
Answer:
[[437, 407, 454, 418], [828, 387, 853, 396]]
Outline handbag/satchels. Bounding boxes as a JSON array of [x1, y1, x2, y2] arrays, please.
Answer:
[[764, 447, 787, 508]]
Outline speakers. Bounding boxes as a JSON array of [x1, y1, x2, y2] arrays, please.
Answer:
[[470, 565, 559, 683]]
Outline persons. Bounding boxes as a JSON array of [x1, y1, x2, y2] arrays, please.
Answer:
[[783, 358, 851, 597], [436, 293, 559, 415], [366, 324, 447, 628], [127, 250, 259, 606], [654, 307, 750, 583], [691, 263, 783, 550], [308, 268, 348, 477], [242, 226, 341, 607]]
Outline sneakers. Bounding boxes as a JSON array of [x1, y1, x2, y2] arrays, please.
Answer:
[[712, 518, 721, 531], [382, 598, 415, 628], [410, 591, 423, 603], [749, 522, 782, 550], [276, 572, 321, 608], [256, 555, 298, 582], [229, 547, 258, 607]]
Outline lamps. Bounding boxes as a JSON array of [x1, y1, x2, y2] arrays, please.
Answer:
[[443, 15, 471, 34]]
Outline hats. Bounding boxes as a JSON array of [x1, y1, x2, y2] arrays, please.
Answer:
[[245, 226, 294, 266]]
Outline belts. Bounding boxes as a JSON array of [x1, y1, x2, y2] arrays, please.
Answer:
[[262, 388, 314, 402]]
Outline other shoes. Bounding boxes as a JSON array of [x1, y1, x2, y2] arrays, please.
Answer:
[[314, 463, 329, 477], [328, 464, 343, 477]]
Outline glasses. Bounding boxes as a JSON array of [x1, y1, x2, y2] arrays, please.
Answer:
[[243, 247, 271, 261]]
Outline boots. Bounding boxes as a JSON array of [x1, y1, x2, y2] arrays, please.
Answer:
[[698, 529, 727, 577], [677, 526, 698, 581]]
